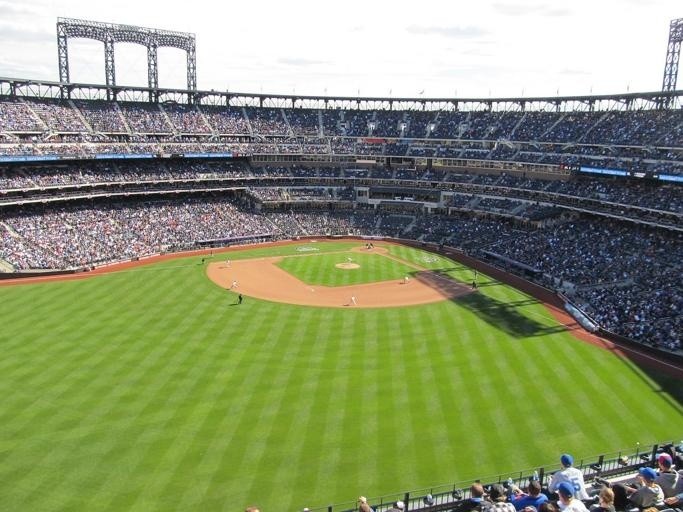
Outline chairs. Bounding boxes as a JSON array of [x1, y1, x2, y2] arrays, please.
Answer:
[[579, 487, 683, 512]]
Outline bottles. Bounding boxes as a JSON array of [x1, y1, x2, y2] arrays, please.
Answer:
[[427, 492, 432, 504], [679, 441, 683, 452], [508, 477, 512, 486], [533, 470, 539, 481]]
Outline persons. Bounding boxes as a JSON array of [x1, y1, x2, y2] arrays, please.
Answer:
[[345, 296, 358, 305], [471, 280, 476, 290], [357, 496, 374, 512], [403, 276, 408, 284], [447, 443, 681, 512], [0, 99, 683, 274], [238, 293, 242, 304], [530, 273, 682, 358], [392, 501, 405, 512], [243, 505, 260, 512], [230, 281, 239, 288], [474, 271, 478, 279]]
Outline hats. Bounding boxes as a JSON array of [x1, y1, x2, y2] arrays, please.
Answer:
[[639, 452, 673, 480], [554, 481, 575, 497], [560, 454, 575, 467]]
